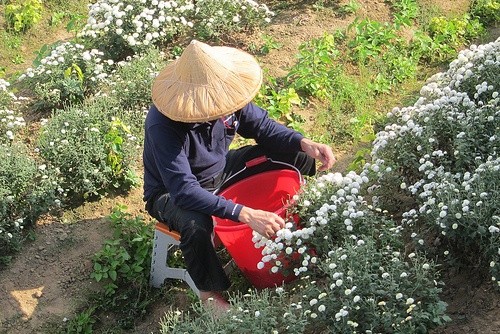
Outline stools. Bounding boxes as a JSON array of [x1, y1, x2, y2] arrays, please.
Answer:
[[149, 222, 238, 310]]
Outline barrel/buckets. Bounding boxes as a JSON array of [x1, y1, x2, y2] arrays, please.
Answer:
[[210, 155, 320, 290]]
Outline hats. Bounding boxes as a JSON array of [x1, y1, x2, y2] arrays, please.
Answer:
[[151, 40, 262, 123]]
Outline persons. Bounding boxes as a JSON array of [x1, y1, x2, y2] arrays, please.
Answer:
[[143, 40, 336, 316]]
